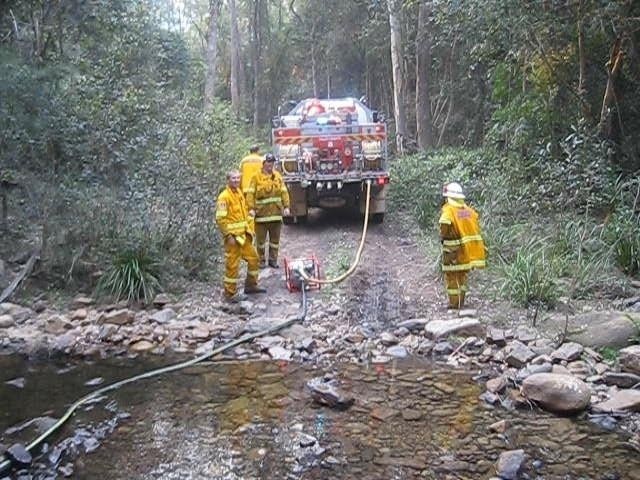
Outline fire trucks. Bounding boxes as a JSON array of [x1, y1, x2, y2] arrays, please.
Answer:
[[271, 97, 391, 225]]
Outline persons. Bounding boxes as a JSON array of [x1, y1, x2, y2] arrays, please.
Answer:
[[239, 145, 291, 268], [214, 169, 267, 303], [438, 182, 486, 310]]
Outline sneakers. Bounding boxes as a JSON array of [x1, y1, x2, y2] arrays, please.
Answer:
[[257, 261, 278, 271], [225, 295, 249, 305], [244, 286, 267, 294]]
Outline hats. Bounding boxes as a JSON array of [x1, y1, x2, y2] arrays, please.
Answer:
[[441, 181, 467, 200], [262, 152, 277, 162]]
